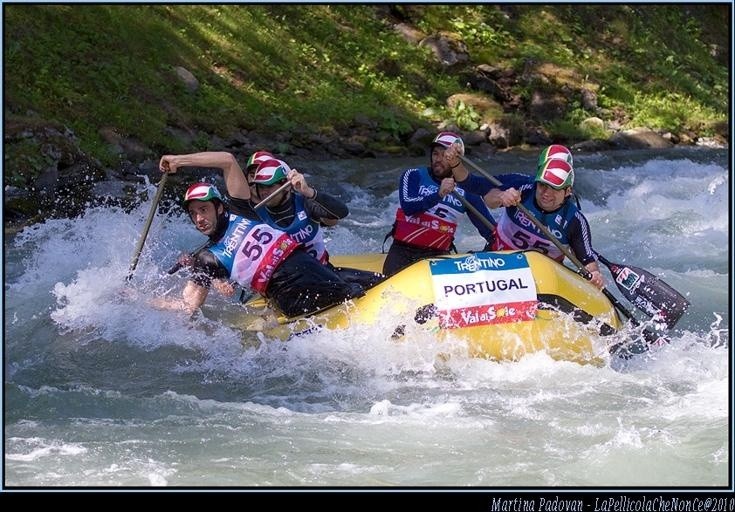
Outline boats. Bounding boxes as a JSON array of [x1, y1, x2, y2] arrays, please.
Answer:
[[245, 248, 627, 373]]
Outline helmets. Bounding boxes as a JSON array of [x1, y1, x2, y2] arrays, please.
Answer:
[[537, 144, 573, 168], [432, 131, 465, 156], [249, 157, 292, 186], [246, 151, 274, 168], [183, 183, 225, 208], [534, 158, 574, 190]]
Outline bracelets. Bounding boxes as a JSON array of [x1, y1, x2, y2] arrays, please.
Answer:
[[451, 160, 463, 169]]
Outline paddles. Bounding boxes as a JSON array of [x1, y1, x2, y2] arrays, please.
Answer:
[[455, 151, 691, 331], [515, 203, 672, 352]]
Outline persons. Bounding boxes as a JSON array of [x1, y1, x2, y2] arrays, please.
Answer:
[[482, 158, 610, 292], [174, 158, 390, 292], [207, 148, 275, 299], [441, 138, 573, 199], [381, 129, 498, 282], [120, 146, 366, 319]]
